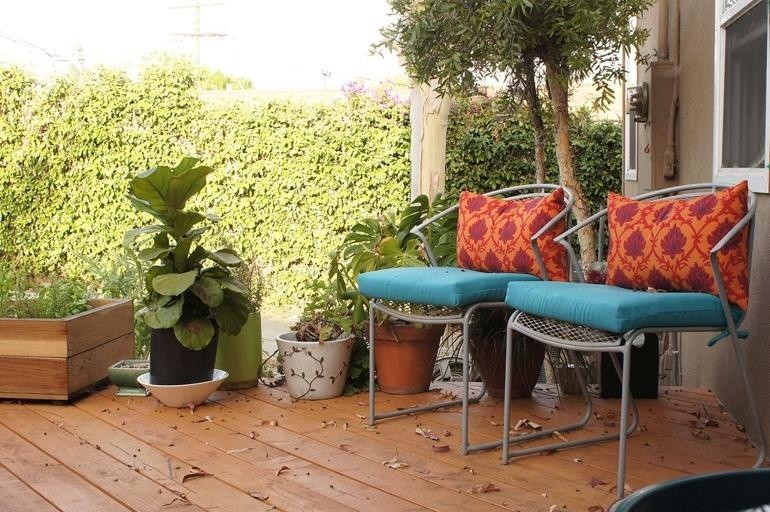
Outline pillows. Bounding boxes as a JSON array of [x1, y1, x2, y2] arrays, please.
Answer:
[[456, 180, 752, 310]]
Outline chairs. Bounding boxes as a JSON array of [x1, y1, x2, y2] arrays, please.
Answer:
[[502, 182, 766, 497], [358, 183, 594, 455]]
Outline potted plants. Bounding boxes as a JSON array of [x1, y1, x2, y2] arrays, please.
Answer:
[[0, 281, 134, 400], [122, 155, 263, 389], [277, 193, 456, 400]]
